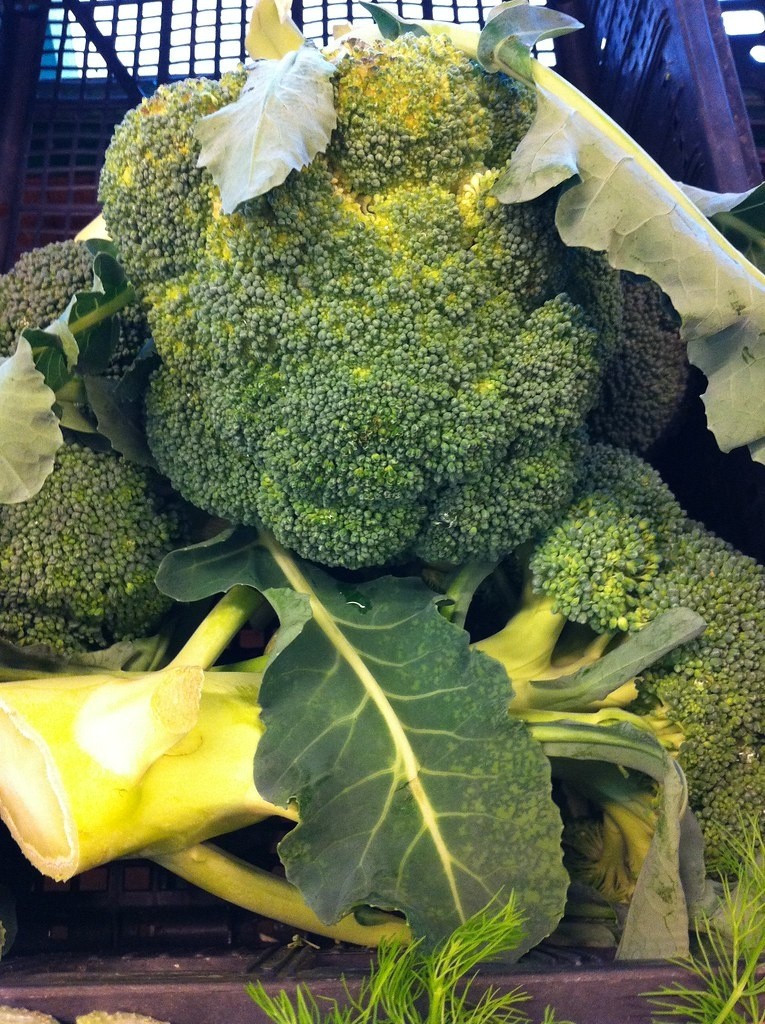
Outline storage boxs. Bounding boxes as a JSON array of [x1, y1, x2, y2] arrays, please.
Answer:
[[0, 1, 765, 1024]]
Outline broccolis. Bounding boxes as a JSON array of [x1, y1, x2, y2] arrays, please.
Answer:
[[2, 7, 763, 978]]
